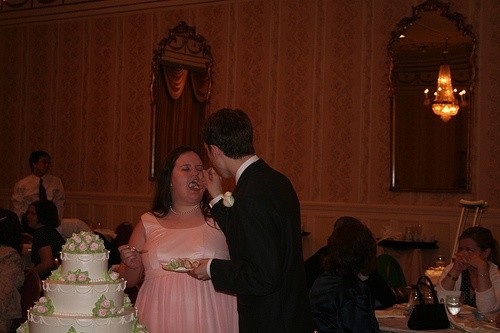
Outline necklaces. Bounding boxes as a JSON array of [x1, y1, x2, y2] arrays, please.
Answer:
[[169, 204, 199, 214]]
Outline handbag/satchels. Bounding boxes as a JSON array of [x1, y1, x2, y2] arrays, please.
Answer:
[[407, 275, 450, 330]]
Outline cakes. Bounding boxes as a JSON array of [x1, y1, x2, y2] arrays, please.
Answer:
[[164, 257, 199, 270], [16, 231, 149, 333]]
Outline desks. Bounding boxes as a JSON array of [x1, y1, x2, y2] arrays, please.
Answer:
[[378, 238, 438, 278]]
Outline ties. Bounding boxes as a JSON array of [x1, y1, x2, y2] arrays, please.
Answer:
[[38, 178, 47, 201]]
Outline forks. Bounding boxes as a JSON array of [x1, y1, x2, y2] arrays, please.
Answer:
[[126, 245, 149, 254]]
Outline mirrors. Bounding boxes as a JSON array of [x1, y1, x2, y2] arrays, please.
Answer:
[[385, 2, 478, 193], [150, 24, 216, 183]]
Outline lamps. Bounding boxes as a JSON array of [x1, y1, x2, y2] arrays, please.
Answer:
[[424, 36, 470, 123]]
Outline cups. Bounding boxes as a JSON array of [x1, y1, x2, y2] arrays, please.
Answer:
[[410, 288, 431, 310], [393, 232, 404, 240]]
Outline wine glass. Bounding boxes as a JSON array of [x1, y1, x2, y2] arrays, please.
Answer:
[[434, 256, 447, 275], [446, 295, 463, 330], [97, 221, 102, 233], [406, 224, 422, 242]]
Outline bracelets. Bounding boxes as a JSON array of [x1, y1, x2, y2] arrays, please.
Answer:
[[447, 273, 458, 280]]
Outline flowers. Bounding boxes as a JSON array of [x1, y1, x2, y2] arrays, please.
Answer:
[[66, 231, 105, 251], [221, 192, 235, 207], [106, 271, 119, 282], [35, 297, 54, 315], [49, 265, 61, 280], [97, 298, 117, 316], [68, 271, 91, 283]]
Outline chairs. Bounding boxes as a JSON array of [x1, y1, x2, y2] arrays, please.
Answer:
[[18, 269, 42, 324], [115, 222, 132, 234], [377, 255, 406, 289]]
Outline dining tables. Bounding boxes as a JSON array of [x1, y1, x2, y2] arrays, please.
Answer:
[[373, 300, 500, 333]]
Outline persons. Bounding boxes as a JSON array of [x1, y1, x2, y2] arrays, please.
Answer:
[[437, 227, 500, 313], [0, 208, 24, 333], [303, 216, 396, 333], [27, 200, 66, 280], [117, 146, 240, 333], [187, 108, 312, 333], [12, 151, 65, 226]]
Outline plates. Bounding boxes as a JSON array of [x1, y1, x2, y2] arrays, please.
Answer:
[[159, 263, 194, 273]]
